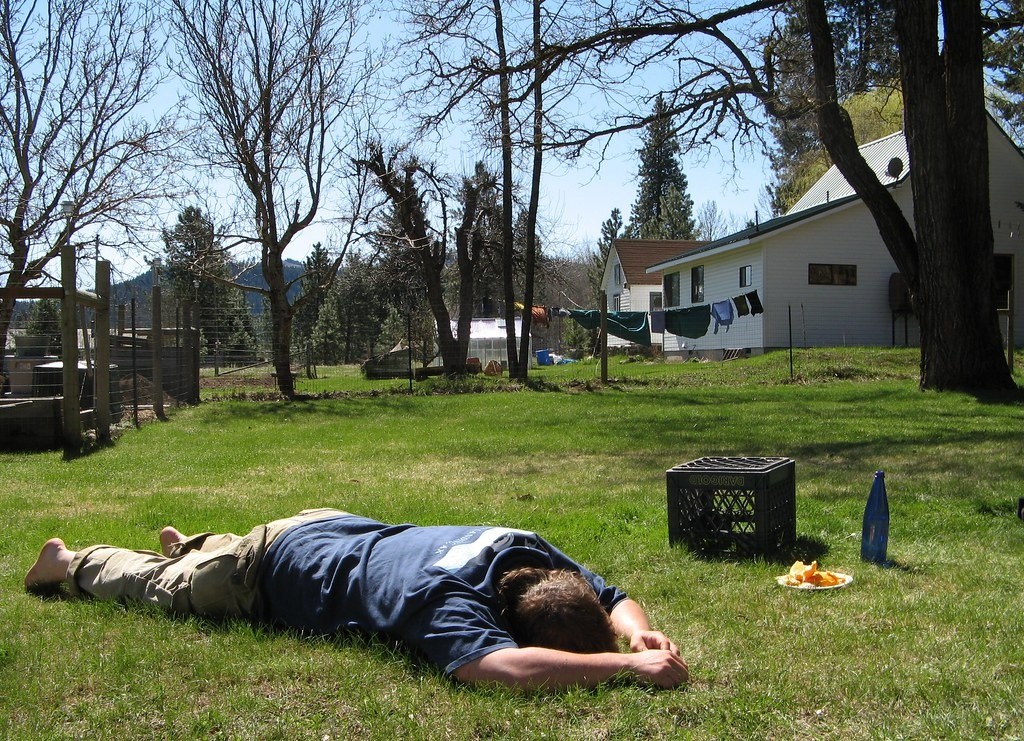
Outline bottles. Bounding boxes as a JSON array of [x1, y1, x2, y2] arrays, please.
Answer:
[[860, 469, 889, 563]]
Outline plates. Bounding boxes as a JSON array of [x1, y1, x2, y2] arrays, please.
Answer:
[[778, 573, 853, 590]]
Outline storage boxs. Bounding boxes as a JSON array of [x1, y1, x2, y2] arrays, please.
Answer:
[[666, 457, 796, 562], [5, 355, 59, 394]]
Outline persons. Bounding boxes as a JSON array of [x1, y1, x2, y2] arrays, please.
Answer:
[[26, 509, 688, 691]]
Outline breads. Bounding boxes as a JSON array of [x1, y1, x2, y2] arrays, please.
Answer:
[[785, 559, 839, 587]]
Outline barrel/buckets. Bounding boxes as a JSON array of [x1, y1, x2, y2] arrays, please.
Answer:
[[536, 350, 550, 365]]
